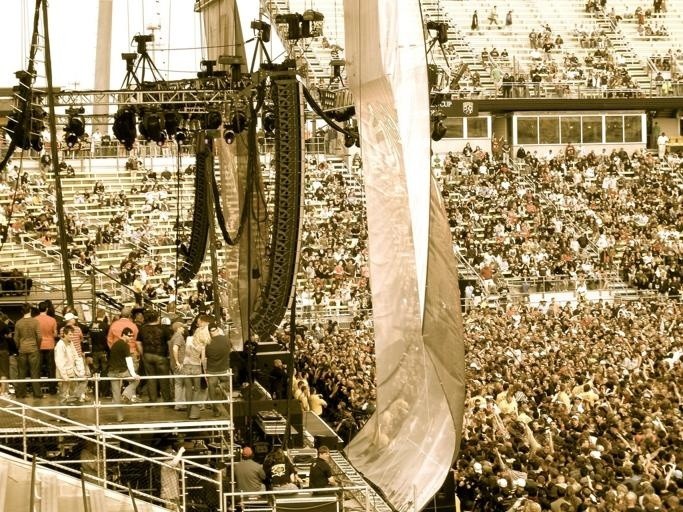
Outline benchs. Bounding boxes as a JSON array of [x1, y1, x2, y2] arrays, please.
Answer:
[[234, 493, 339, 512]]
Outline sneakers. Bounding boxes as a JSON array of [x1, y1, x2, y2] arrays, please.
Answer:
[[120, 395, 143, 404]]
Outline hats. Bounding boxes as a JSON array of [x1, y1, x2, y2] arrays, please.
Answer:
[[208, 323, 218, 332], [172, 321, 189, 331], [242, 447, 252, 457], [63, 313, 78, 322]]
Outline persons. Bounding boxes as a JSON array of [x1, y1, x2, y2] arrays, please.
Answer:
[[2, 129, 230, 419], [470, 1, 682, 97], [47, 435, 336, 510], [257, 125, 377, 444], [312, 34, 340, 85], [433, 134, 682, 511]]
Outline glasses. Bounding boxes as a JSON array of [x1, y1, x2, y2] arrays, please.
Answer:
[[127, 334, 134, 337]]
